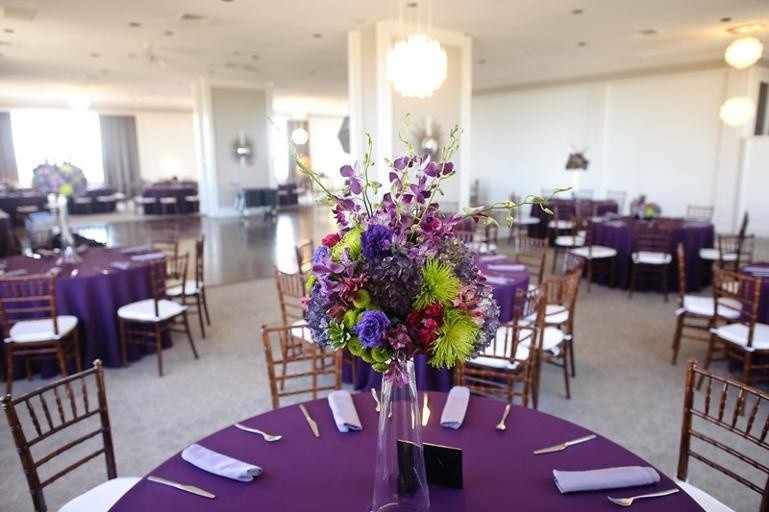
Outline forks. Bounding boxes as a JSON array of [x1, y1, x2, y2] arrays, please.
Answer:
[[233, 422, 283, 442], [371, 387, 383, 413], [607, 487, 680, 506], [387, 400, 393, 417], [496, 403, 511, 431]]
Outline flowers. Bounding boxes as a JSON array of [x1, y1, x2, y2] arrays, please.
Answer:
[[262, 116, 571, 388]]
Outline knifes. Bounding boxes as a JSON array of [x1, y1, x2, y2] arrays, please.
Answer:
[[0, 240, 164, 280], [532, 434, 596, 455], [410, 402, 415, 430], [147, 475, 215, 498], [422, 392, 431, 427], [298, 403, 320, 437]]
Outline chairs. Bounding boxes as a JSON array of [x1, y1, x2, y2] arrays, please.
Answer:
[[2, 356, 140, 509], [0, 273, 88, 398], [627, 218, 678, 301], [259, 232, 584, 411], [468, 179, 498, 249], [671, 243, 744, 366], [696, 269, 768, 416], [116, 252, 201, 382], [713, 232, 756, 300], [502, 193, 618, 294], [163, 234, 213, 339], [698, 211, 758, 294]]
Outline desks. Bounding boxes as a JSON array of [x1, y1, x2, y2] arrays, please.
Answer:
[[585, 214, 716, 298], [2, 242, 173, 381], [738, 262, 767, 326]]
